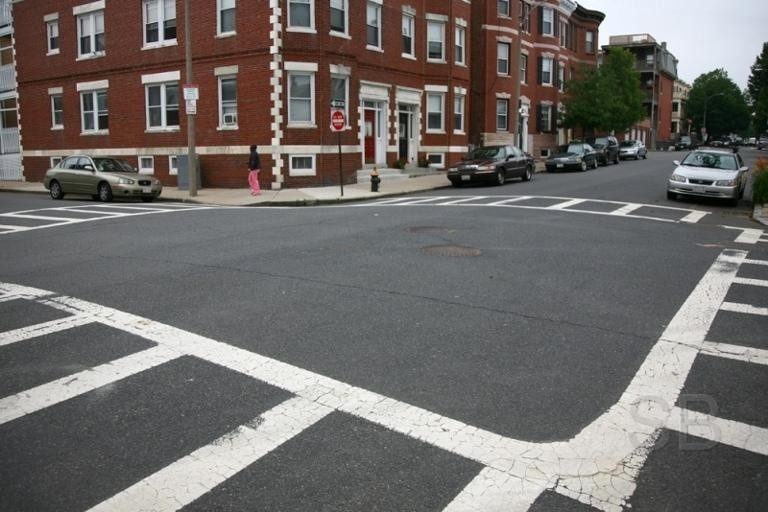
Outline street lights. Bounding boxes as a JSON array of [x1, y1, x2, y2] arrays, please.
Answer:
[[703, 93, 725, 142]]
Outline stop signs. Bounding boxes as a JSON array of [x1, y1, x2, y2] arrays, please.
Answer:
[[330, 109, 345, 131]]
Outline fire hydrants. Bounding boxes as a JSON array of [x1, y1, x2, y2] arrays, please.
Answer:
[[370, 167, 381, 192]]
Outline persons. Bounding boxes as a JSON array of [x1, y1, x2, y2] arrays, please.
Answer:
[[246, 142, 260, 197]]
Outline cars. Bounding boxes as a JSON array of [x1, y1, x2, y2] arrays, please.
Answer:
[[665, 149, 748, 208], [44, 153, 161, 201], [544, 135, 648, 171], [673, 134, 768, 152], [448, 144, 537, 185]]
